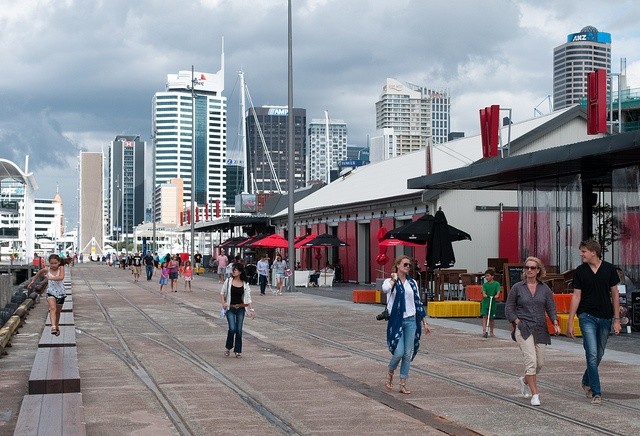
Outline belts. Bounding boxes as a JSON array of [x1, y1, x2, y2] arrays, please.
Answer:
[[230, 303, 249, 309]]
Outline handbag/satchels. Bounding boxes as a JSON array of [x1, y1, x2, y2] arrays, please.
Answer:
[[285, 269, 293, 277], [167, 262, 171, 274]]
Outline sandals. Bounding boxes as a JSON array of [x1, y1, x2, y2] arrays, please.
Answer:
[[398, 382, 411, 394], [385, 371, 394, 390]]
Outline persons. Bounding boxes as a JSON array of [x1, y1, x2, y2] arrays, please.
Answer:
[[273, 253, 290, 295], [66, 251, 70, 266], [102, 252, 134, 269], [257, 254, 270, 296], [73, 252, 78, 264], [179, 262, 184, 277], [209, 256, 217, 273], [382, 255, 430, 394], [159, 262, 169, 293], [164, 253, 170, 263], [28, 254, 66, 337], [194, 250, 203, 274], [153, 253, 159, 268], [176, 253, 181, 267], [226, 252, 241, 262], [183, 260, 192, 291], [294, 261, 301, 270], [143, 251, 154, 281], [616, 266, 634, 297], [88, 253, 93, 261], [504, 258, 562, 406], [80, 253, 83, 263], [97, 255, 100, 261], [33, 252, 39, 272], [131, 252, 142, 282], [569, 239, 622, 404], [218, 250, 229, 283], [481, 269, 502, 338], [326, 261, 333, 268], [221, 262, 256, 360], [167, 255, 179, 292]]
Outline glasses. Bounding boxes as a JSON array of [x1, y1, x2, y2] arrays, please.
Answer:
[[525, 266, 536, 269], [404, 263, 412, 267]]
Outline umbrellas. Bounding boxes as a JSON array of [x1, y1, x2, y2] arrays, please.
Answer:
[[240, 234, 270, 261], [215, 238, 232, 248], [378, 238, 427, 279], [225, 236, 247, 258], [251, 234, 288, 257], [299, 233, 351, 266], [294, 233, 326, 270], [426, 206, 455, 302], [294, 234, 312, 270], [294, 235, 303, 242], [384, 209, 472, 244], [376, 224, 389, 279]]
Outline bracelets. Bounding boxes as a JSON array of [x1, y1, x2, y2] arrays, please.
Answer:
[[554, 324, 560, 326], [614, 317, 621, 320], [423, 322, 429, 326], [249, 308, 255, 311]]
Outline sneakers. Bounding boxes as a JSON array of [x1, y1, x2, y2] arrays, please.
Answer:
[[519, 377, 530, 398], [530, 394, 541, 406], [51, 326, 57, 334], [582, 385, 593, 398], [591, 395, 601, 406], [55, 329, 60, 336], [236, 353, 242, 359], [224, 347, 230, 356]]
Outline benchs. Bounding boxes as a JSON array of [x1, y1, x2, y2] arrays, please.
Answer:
[[49, 301, 73, 312], [12, 392, 87, 436], [29, 346, 80, 394], [38, 326, 76, 347], [63, 272, 72, 294], [65, 294, 72, 301], [45, 312, 75, 326]]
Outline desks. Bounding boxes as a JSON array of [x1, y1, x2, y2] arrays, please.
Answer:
[[434, 269, 467, 299]]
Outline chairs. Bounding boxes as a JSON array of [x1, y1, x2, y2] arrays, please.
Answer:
[[458, 273, 471, 301], [294, 270, 310, 287], [318, 272, 335, 287], [560, 269, 576, 294], [447, 272, 459, 300]]
